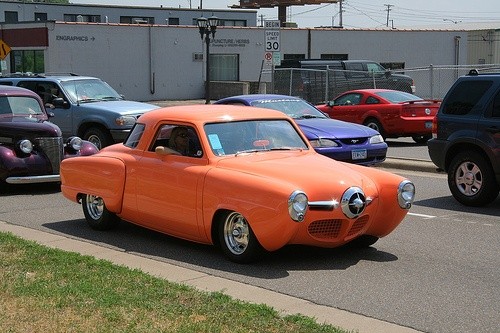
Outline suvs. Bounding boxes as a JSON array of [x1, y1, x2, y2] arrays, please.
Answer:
[[0, 76, 165, 150], [272, 60, 414, 109], [428, 69, 500, 207]]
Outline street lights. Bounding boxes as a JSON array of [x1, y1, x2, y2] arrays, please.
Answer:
[[197, 15, 219, 106]]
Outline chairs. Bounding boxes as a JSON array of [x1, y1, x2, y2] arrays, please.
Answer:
[[74, 84, 89, 100]]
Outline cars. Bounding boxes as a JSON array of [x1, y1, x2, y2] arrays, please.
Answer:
[[0, 85, 101, 195], [56, 104, 416, 263], [208, 94, 390, 168], [312, 89, 441, 144]]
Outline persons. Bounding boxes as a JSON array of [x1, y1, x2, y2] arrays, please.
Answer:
[[171, 127, 195, 156], [227, 127, 254, 150]]
